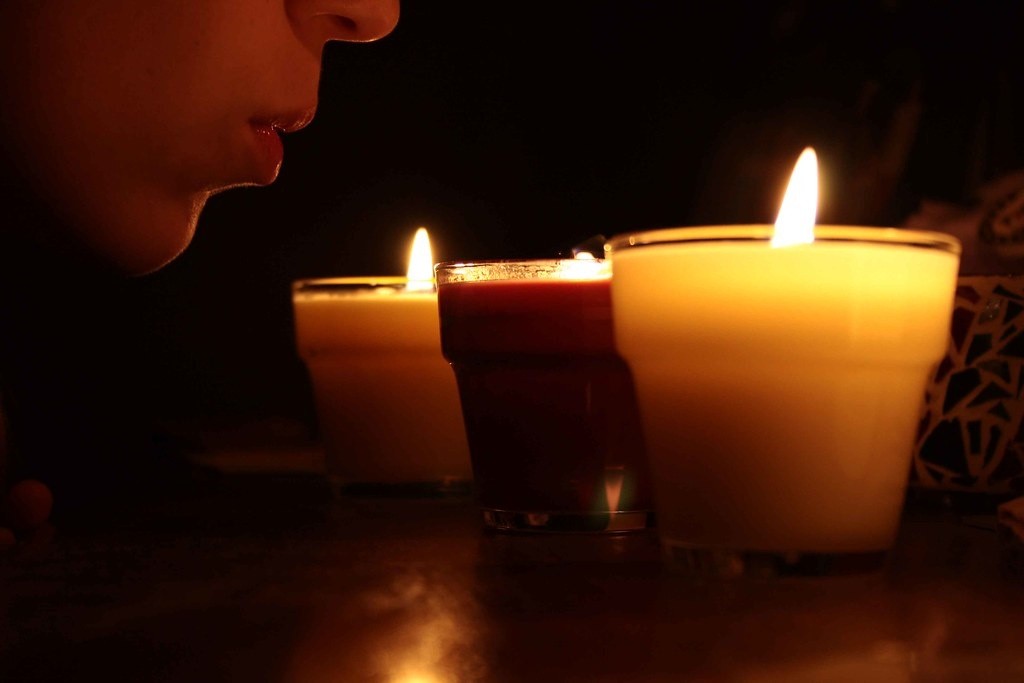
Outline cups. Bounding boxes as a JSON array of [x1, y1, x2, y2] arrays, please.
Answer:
[[437, 257, 649, 535], [292, 275, 474, 497], [604, 224, 962, 574]]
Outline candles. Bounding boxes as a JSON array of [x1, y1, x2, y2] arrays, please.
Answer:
[[440, 251, 650, 534], [603, 147, 963, 580], [292, 225, 472, 512]]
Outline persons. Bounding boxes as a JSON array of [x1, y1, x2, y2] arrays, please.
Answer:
[[0, 0, 404, 277]]
